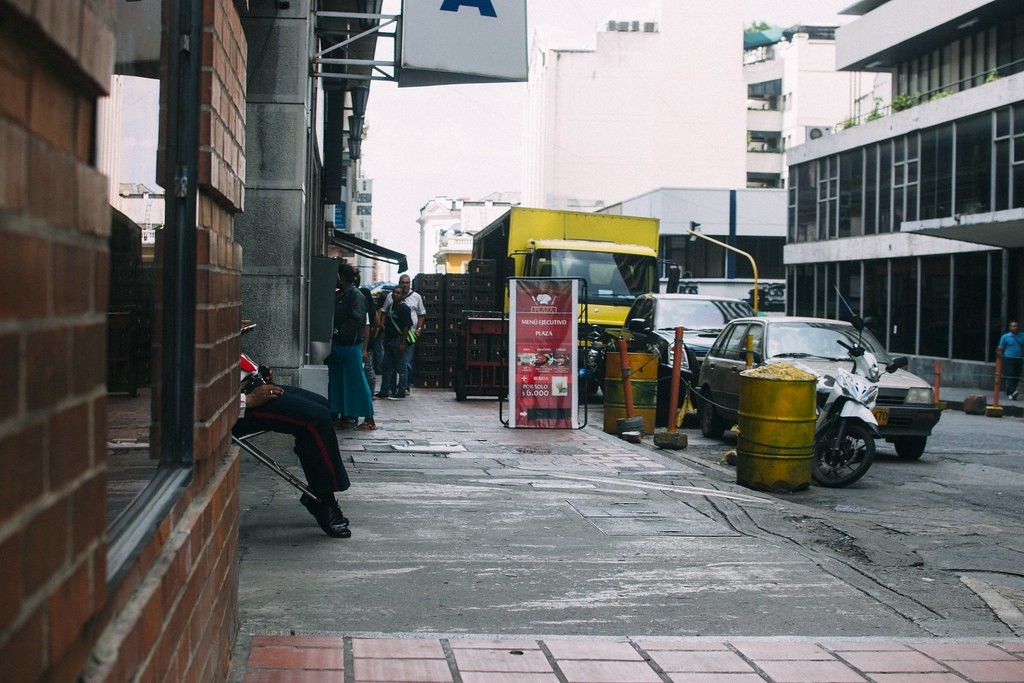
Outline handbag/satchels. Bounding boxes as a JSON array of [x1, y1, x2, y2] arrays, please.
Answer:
[[406, 329, 418, 343]]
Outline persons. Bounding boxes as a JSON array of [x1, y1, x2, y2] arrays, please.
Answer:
[[326, 264, 376, 430], [231, 320, 351, 540], [353, 268, 426, 400], [996, 320, 1023, 399]]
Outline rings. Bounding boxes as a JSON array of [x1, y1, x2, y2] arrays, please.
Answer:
[[270, 390, 273, 395]]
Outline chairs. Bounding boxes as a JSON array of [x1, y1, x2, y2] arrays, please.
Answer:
[[611, 265, 632, 291], [230, 373, 317, 505], [541, 264, 557, 277]]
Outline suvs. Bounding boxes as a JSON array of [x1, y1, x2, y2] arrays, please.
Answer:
[[617, 293, 758, 417]]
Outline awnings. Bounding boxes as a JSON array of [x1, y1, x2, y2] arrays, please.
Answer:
[[333, 229, 407, 273]]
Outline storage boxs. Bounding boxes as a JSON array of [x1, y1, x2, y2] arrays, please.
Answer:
[[411, 258, 500, 388]]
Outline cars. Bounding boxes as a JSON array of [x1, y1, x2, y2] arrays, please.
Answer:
[[695, 316, 947, 460]]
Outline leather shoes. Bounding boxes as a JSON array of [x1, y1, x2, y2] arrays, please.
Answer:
[[300, 486, 352, 538]]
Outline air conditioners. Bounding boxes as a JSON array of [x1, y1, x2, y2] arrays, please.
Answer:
[[805, 125, 832, 143]]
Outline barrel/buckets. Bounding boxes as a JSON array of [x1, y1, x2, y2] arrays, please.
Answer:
[[604, 352, 660, 435], [736, 369, 819, 493]]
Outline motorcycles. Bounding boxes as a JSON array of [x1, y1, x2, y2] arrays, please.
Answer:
[[814, 316, 909, 488]]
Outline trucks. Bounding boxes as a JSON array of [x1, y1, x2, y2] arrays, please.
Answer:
[[472, 207, 680, 396]]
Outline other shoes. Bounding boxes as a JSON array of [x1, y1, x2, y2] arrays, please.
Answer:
[[393, 391, 406, 398], [375, 392, 389, 397], [404, 388, 411, 396], [387, 388, 398, 396], [335, 420, 376, 430], [1008, 391, 1019, 401]]
